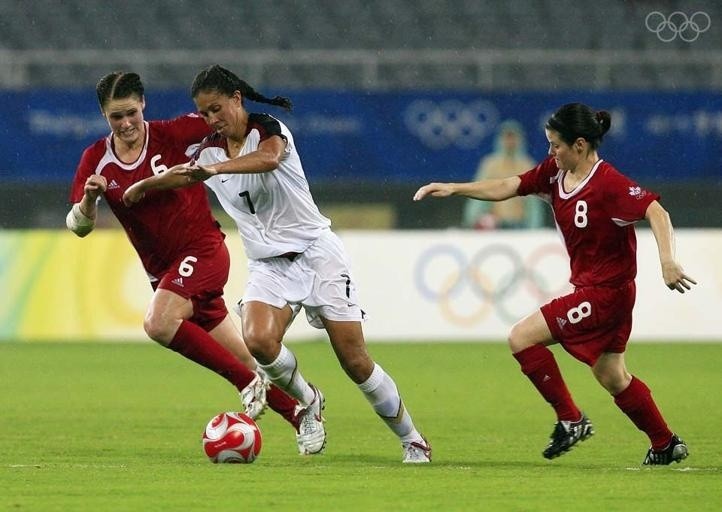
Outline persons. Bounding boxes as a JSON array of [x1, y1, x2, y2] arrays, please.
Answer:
[[459, 115, 550, 228], [413, 102, 699, 467], [123, 63, 432, 464], [63, 72, 310, 458]]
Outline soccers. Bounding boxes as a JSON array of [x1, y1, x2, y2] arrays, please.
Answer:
[[203, 412, 261, 464]]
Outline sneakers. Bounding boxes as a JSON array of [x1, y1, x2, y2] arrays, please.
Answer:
[[295, 383, 326, 456], [402, 435, 432, 463], [642, 434, 689, 467], [239, 370, 271, 422], [543, 413, 594, 460]]
